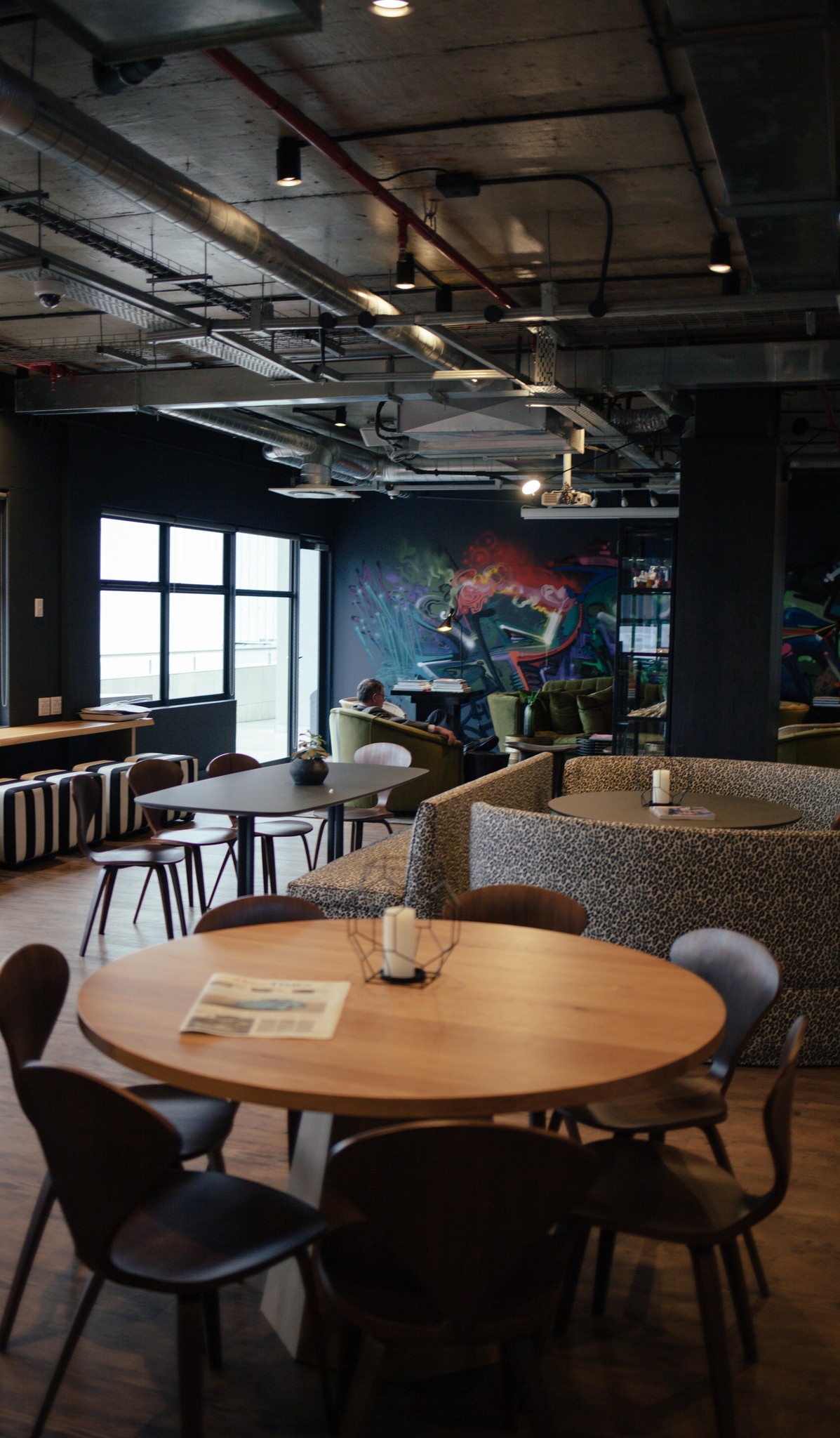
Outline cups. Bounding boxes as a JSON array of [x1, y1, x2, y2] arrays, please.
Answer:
[[653, 770, 672, 804], [381, 905, 417, 978]]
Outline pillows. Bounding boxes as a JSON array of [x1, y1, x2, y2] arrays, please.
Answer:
[[516, 687, 564, 730], [575, 686, 613, 734], [548, 688, 592, 735]]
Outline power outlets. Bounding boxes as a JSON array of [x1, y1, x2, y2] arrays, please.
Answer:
[[48, 696, 63, 716], [37, 697, 50, 716]]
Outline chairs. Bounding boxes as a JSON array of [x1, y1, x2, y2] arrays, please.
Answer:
[[338, 695, 407, 721], [313, 741, 413, 870], [125, 758, 240, 927], [206, 750, 314, 911], [65, 770, 191, 959], [0, 883, 814, 1438]]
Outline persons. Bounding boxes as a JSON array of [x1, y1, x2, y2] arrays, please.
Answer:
[[351, 678, 499, 757]]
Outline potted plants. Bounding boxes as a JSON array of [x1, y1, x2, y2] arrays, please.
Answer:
[[510, 687, 546, 737], [285, 727, 332, 785]]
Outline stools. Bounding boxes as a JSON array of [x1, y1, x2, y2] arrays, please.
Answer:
[[0, 750, 201, 865]]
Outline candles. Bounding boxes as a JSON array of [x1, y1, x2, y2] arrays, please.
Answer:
[[650, 768, 674, 806], [380, 900, 419, 981]]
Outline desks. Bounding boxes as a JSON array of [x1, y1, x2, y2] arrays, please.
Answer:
[[69, 913, 736, 1360], [0, 715, 157, 756], [390, 686, 484, 733]]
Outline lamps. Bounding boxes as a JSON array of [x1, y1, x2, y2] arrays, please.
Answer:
[[706, 239, 733, 275], [272, 128, 307, 190], [391, 249, 418, 293], [330, 403, 349, 428]]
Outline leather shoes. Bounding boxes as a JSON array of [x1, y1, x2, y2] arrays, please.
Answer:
[[463, 735, 499, 753]]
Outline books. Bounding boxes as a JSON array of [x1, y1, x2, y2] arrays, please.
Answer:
[[392, 678, 472, 692], [648, 806, 715, 820]]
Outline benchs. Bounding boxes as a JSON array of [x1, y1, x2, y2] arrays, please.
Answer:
[[561, 752, 840, 829], [465, 798, 840, 1067], [285, 747, 558, 917]]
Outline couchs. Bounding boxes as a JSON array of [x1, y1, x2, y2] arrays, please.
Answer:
[[327, 706, 465, 813], [486, 675, 615, 751]]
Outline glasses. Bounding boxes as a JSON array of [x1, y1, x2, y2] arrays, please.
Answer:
[[374, 693, 388, 700]]
